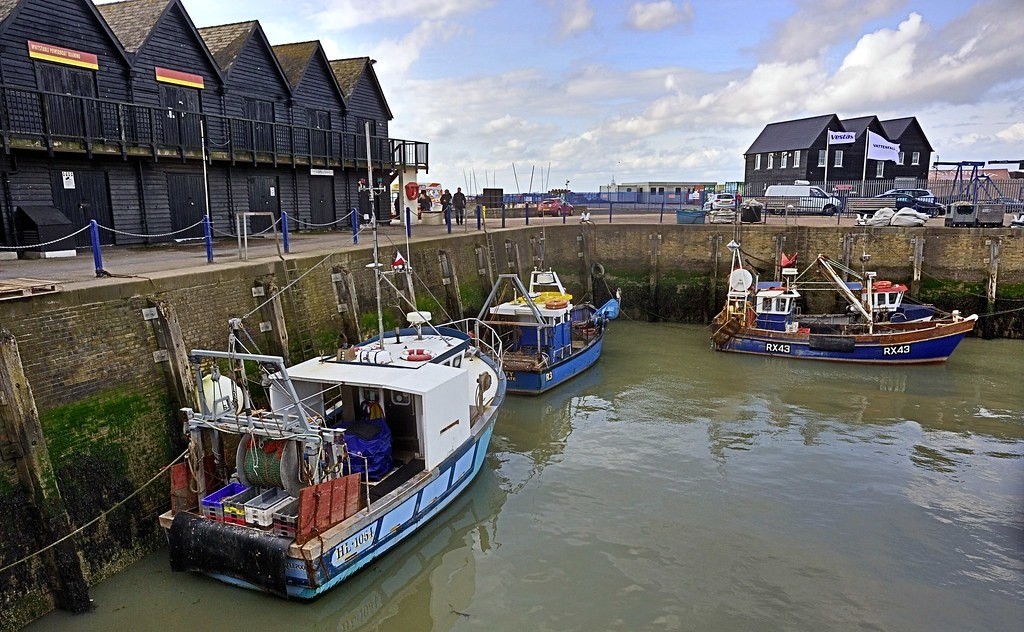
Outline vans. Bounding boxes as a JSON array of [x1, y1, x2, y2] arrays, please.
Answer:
[[764, 181, 842, 216]]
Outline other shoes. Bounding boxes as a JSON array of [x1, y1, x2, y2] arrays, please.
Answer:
[[457, 222, 463, 225]]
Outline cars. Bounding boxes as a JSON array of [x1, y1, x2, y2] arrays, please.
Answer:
[[538, 197, 575, 217], [859, 193, 946, 219], [702, 193, 735, 210], [989, 196, 1023, 213]]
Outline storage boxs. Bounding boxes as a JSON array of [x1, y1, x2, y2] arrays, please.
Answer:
[[200, 481, 297, 538]]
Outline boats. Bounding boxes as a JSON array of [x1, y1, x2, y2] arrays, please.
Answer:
[[493, 364, 609, 464], [711, 219, 981, 364], [161, 120, 508, 601], [164, 460, 510, 632], [712, 349, 964, 433], [470, 223, 607, 397]]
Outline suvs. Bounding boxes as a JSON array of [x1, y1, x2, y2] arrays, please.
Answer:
[[876, 189, 934, 204]]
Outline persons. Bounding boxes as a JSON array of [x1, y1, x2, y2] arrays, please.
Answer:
[[440, 189, 452, 225], [394, 190, 432, 220], [452, 187, 466, 225]]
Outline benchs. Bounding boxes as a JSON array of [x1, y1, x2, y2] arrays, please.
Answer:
[[847, 197, 898, 218], [755, 197, 800, 215]]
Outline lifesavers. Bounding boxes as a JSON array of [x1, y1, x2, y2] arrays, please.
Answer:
[[400, 349, 436, 361], [546, 298, 568, 309]]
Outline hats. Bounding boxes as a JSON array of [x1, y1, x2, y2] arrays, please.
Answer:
[[444, 189, 449, 193]]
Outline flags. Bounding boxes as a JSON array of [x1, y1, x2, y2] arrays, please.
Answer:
[[829, 131, 856, 144], [867, 130, 900, 165]]
